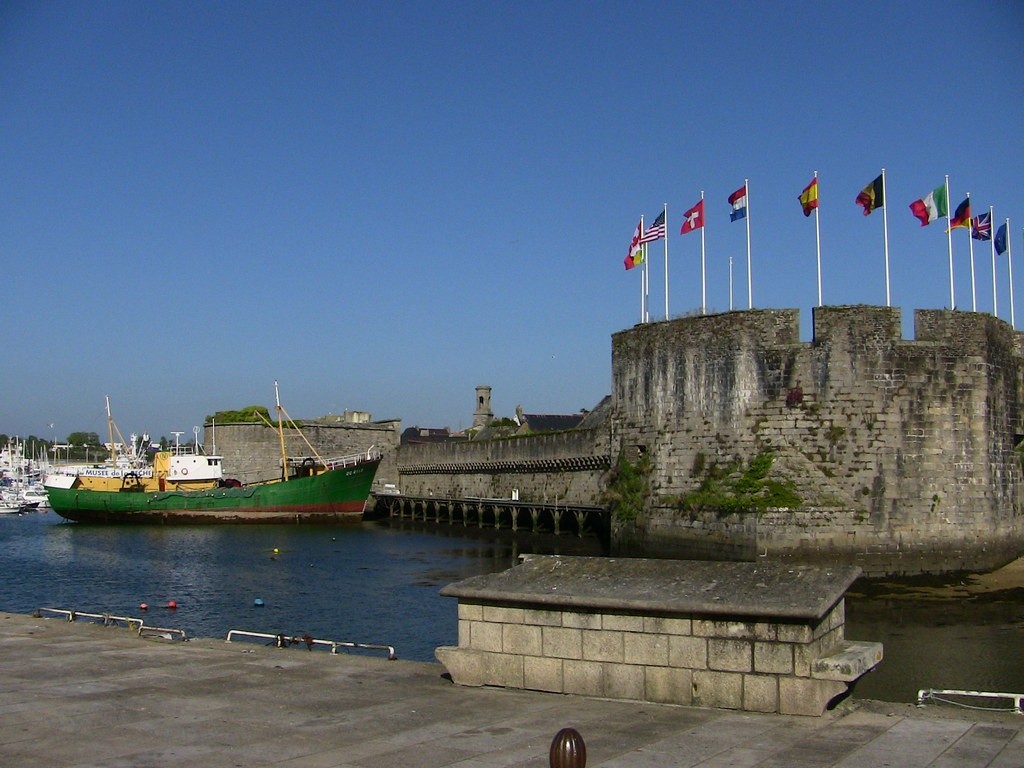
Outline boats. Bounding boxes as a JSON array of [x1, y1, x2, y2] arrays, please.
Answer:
[[0, 393, 222, 514], [43, 381, 386, 524]]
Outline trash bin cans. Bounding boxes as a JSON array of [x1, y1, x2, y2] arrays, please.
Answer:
[[512, 489, 519, 501]]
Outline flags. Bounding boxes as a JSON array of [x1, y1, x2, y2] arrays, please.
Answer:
[[994, 221, 1007, 256], [623, 221, 645, 271], [908, 183, 948, 227], [727, 185, 747, 223], [968, 212, 991, 241], [944, 198, 971, 234], [639, 210, 666, 244], [856, 174, 883, 216], [680, 199, 705, 235], [797, 177, 819, 217]]
